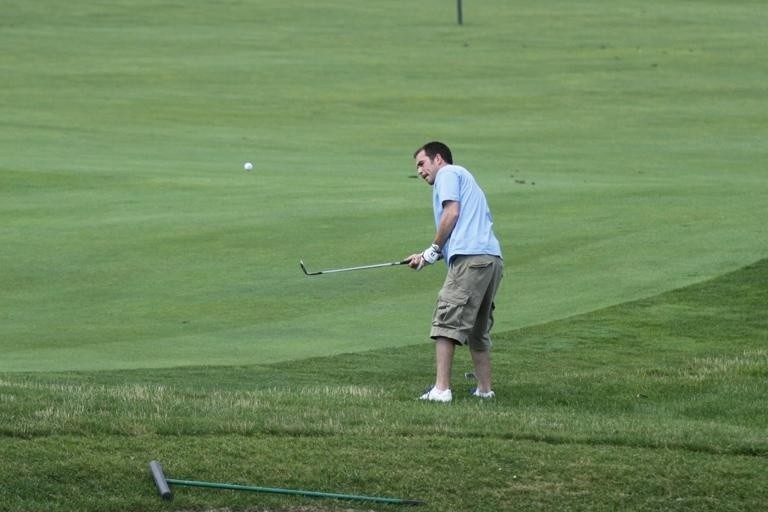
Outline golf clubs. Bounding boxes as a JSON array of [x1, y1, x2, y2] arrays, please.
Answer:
[[300, 259, 416, 276]]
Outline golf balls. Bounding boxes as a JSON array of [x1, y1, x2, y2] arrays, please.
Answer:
[[244, 160, 252, 170]]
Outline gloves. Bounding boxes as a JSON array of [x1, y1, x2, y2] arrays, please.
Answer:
[[414, 244, 442, 272]]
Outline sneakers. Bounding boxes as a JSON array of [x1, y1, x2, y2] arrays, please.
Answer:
[[419, 385, 451, 403], [472, 389, 495, 398]]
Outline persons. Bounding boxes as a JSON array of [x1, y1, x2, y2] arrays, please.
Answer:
[[400, 140, 506, 403]]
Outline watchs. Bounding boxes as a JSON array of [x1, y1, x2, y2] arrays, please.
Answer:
[[430, 243, 442, 254]]
